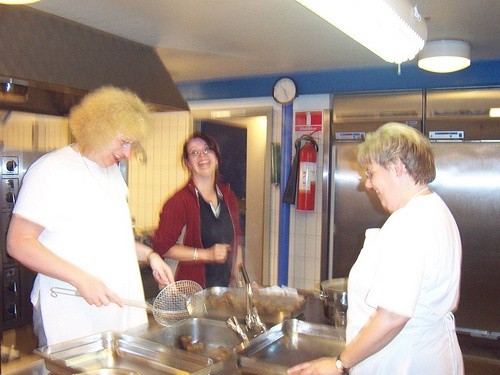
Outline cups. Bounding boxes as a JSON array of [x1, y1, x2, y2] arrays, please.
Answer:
[[333, 307, 348, 339]]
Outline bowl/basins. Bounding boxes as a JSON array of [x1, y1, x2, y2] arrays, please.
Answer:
[[317, 277, 348, 325]]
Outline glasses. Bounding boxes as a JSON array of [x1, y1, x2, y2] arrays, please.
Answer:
[[363, 163, 380, 176], [119, 139, 140, 149], [188, 147, 213, 156]]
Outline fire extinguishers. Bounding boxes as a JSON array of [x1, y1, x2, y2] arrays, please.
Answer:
[[282, 129, 320, 211]]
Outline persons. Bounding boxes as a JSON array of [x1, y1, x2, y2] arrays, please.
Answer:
[[286, 122, 464, 375], [152, 132, 244, 296], [5, 87, 177, 348]]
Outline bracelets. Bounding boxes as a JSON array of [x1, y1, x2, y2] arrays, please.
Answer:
[[193, 248, 199, 263], [147, 250, 156, 266]]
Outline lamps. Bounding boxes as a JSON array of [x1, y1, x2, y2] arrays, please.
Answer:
[[417, 39, 471, 73], [296, 0, 427, 75]]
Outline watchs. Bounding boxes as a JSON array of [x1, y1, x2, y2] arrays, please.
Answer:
[[336, 352, 350, 375]]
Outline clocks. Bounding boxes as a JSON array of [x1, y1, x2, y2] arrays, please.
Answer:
[[272, 76, 298, 105]]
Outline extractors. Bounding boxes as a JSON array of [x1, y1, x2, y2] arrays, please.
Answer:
[[0, 2, 190, 118]]
